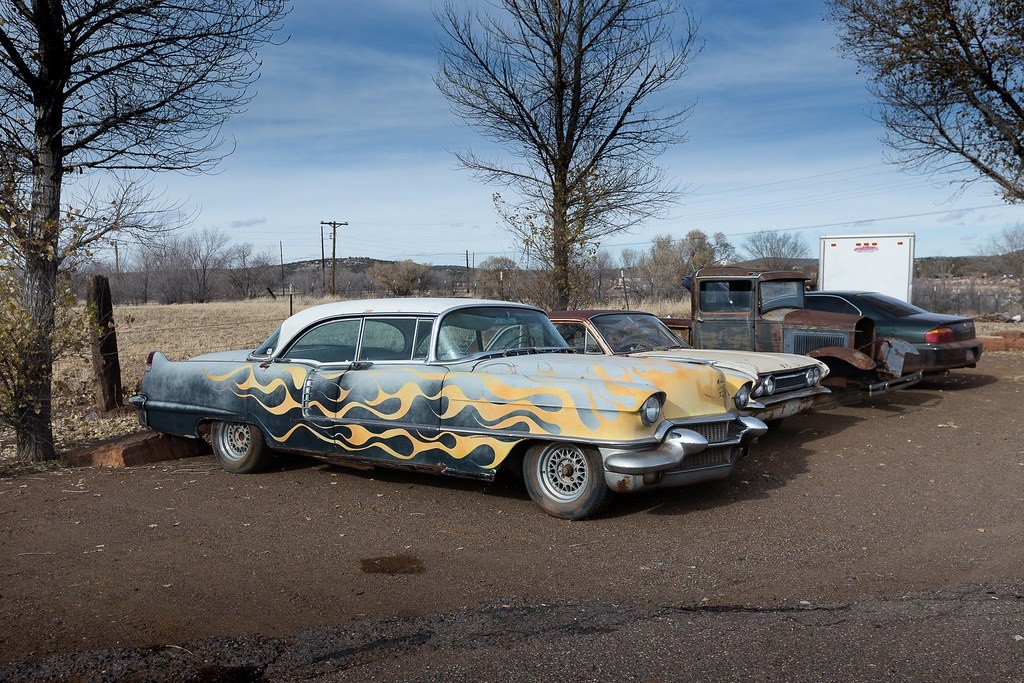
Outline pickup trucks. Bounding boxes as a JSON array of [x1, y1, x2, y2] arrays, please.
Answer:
[[658, 268, 925, 398]]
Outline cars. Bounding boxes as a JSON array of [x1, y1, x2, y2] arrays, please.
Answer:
[[760, 289, 983, 377], [464, 311, 832, 421], [129, 297, 768, 520]]
